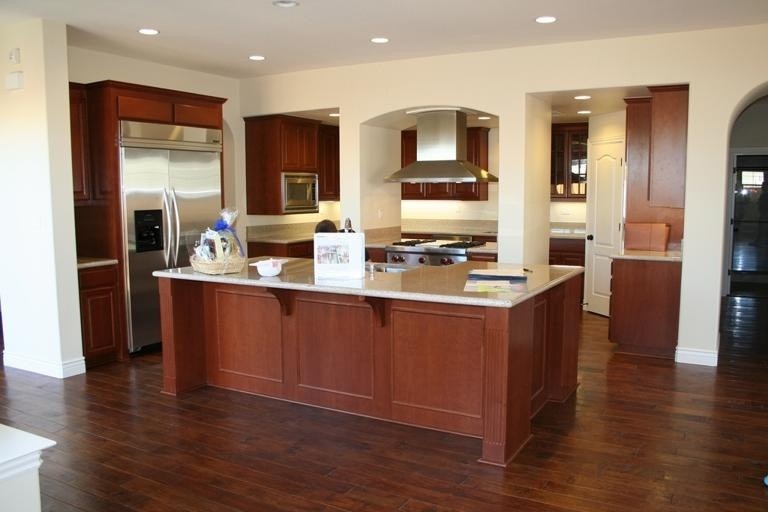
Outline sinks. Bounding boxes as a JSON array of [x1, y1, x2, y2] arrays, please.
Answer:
[[373, 265, 409, 272]]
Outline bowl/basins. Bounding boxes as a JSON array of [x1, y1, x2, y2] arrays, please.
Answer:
[[256, 260, 282, 277]]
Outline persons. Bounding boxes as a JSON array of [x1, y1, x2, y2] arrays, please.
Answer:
[[315, 220, 372, 262], [748, 181, 768, 245]]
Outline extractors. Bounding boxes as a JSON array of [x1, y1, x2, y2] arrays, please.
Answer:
[[382, 109, 498, 184]]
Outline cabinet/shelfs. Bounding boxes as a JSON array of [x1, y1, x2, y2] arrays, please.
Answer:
[[241, 114, 339, 216], [401, 126, 490, 201], [608, 83, 690, 361], [549, 238, 585, 303], [248, 242, 314, 259]]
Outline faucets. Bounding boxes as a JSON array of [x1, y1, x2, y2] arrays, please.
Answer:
[[344, 217, 351, 228]]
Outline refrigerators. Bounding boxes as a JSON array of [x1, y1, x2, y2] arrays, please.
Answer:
[[119, 119, 224, 353]]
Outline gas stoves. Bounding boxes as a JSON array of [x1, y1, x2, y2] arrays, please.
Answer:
[[385, 237, 484, 266]]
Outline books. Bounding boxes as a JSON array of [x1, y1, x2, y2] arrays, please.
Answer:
[[463, 279, 511, 293], [468, 269, 526, 282]]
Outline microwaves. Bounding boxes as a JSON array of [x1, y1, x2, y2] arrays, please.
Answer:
[[280, 172, 319, 213]]
